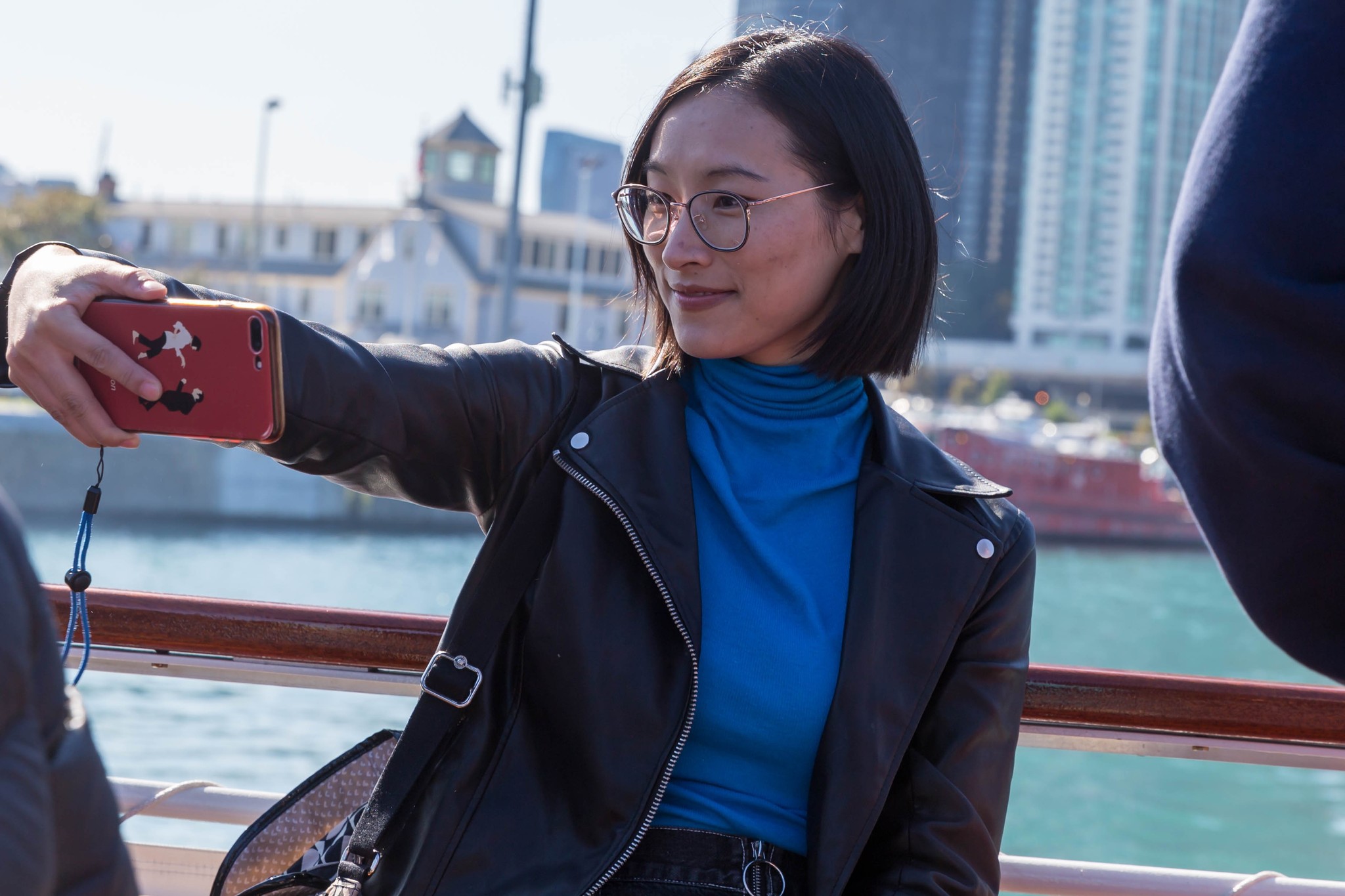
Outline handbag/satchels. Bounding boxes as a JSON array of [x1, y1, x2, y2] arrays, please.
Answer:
[[209, 729, 412, 896]]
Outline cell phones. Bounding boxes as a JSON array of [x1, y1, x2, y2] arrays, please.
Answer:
[[74, 297, 285, 445]]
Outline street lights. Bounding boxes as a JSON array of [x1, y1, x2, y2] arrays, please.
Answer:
[[245, 97, 284, 295]]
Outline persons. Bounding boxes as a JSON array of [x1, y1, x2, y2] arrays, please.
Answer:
[[1143, 0, 1345, 683], [0, 0, 1036, 896]]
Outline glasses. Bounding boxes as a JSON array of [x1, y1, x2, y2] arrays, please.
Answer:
[[611, 183, 836, 253]]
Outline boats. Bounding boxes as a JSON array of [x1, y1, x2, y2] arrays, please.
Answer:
[[928, 423, 1210, 548]]
